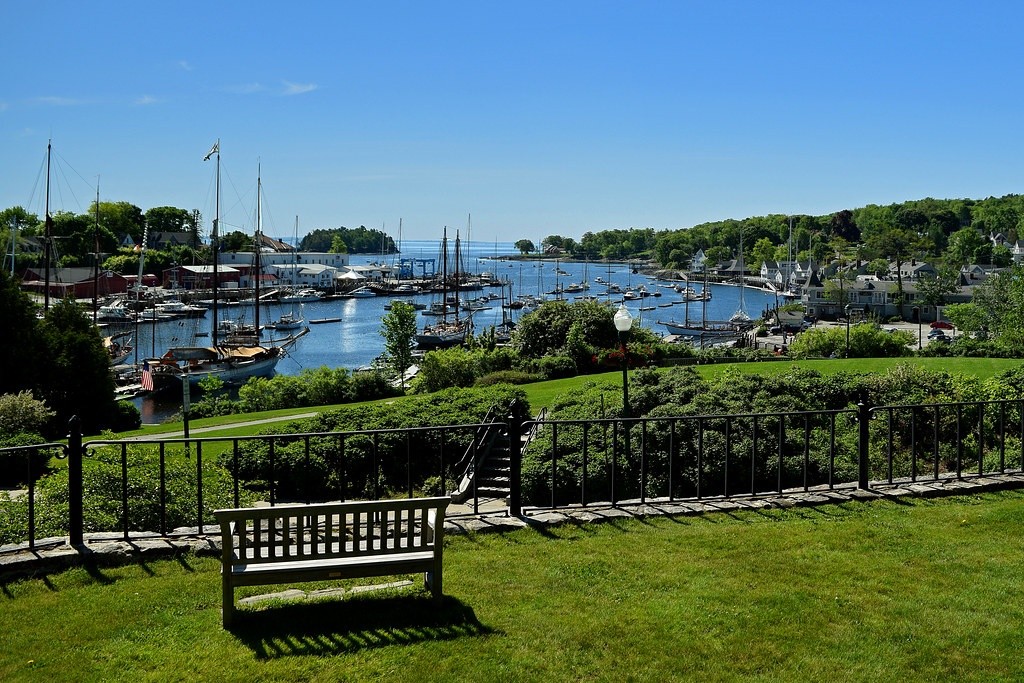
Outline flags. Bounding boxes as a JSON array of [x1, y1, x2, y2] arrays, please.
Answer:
[[142, 360, 153, 391], [203, 137, 220, 161]]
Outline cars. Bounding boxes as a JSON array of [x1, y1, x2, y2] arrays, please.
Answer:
[[927, 330, 951, 345], [930, 321, 954, 330]]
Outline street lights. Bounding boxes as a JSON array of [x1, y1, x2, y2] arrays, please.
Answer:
[[989, 255, 994, 288], [844, 302, 852, 356], [613, 299, 633, 462]]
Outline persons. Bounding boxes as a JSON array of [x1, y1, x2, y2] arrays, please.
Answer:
[[830, 351, 837, 358]]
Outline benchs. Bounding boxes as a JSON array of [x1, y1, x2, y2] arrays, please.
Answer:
[[213, 496, 453, 629]]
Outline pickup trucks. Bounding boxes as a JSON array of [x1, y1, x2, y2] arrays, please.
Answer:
[[770, 323, 801, 335]]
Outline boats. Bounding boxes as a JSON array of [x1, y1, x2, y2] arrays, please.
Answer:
[[555, 287, 562, 294], [384, 298, 427, 311], [422, 303, 451, 316], [567, 283, 583, 292], [623, 291, 637, 300], [654, 289, 662, 297], [447, 296, 463, 307], [639, 288, 650, 297], [460, 300, 484, 308]]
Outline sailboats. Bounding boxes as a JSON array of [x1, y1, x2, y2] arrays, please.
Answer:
[[3, 137, 494, 398], [415, 225, 474, 348], [489, 284, 523, 350], [656, 229, 755, 339], [472, 257, 489, 303]]
